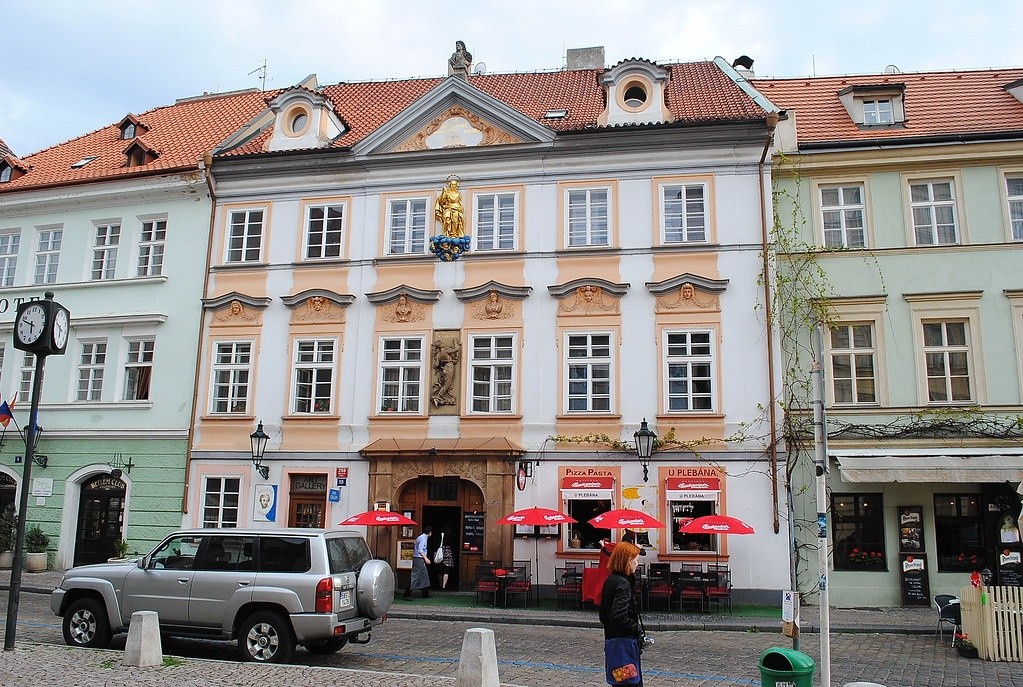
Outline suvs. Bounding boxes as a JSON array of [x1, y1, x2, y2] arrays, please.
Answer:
[[49, 528, 394, 663]]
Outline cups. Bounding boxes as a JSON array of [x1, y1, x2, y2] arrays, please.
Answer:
[[656, 572, 660, 576], [514, 569, 518, 574], [464, 543, 470, 549], [689, 573, 695, 577]]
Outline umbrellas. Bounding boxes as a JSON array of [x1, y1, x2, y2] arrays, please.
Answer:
[[679, 514, 755, 591], [338, 509, 418, 556], [588, 508, 665, 529], [494, 506, 578, 606]]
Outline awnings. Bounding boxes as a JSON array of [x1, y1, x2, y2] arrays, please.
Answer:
[[834, 455, 1023, 482]]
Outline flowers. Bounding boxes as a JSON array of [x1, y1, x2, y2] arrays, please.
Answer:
[[848, 546, 882, 564], [939, 553, 977, 568], [952, 633, 974, 648]]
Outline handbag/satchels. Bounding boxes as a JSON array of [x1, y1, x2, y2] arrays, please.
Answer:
[[434, 547, 444, 564], [604, 638, 640, 685]]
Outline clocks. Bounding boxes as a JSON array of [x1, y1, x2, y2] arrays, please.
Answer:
[[52, 308, 69, 351], [17, 303, 47, 346]]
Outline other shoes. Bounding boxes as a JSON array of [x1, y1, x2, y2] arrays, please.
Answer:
[[402, 596, 413, 601], [439, 585, 445, 591]]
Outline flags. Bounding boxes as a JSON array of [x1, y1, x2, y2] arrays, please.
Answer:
[[0, 401, 14, 421]]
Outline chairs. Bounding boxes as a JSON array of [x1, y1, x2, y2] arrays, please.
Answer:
[[474, 559, 532, 609], [933, 594, 961, 648], [554, 561, 733, 614], [238, 543, 253, 570]]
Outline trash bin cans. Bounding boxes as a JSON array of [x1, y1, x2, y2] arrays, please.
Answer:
[[758, 647, 816, 687]]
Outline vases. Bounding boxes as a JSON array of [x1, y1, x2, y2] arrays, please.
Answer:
[[940, 565, 968, 571], [848, 560, 881, 571], [957, 647, 977, 658]]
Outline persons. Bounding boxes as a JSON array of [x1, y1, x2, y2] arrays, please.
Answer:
[[437, 180, 465, 237], [433, 527, 454, 591], [599, 534, 646, 556], [402, 526, 431, 600], [450, 40, 472, 71], [599, 541, 645, 687]]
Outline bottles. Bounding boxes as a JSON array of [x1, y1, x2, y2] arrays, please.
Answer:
[[704, 545, 708, 551], [571, 538, 610, 549], [402, 526, 412, 537]]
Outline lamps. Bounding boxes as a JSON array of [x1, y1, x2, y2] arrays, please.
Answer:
[[250, 421, 270, 480], [23, 423, 48, 469], [633, 417, 657, 482]]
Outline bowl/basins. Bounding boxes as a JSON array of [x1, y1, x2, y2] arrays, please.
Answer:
[[471, 547, 478, 552]]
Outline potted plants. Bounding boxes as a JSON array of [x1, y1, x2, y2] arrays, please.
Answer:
[[0, 502, 18, 569], [24, 524, 50, 573], [108, 539, 129, 563]]
